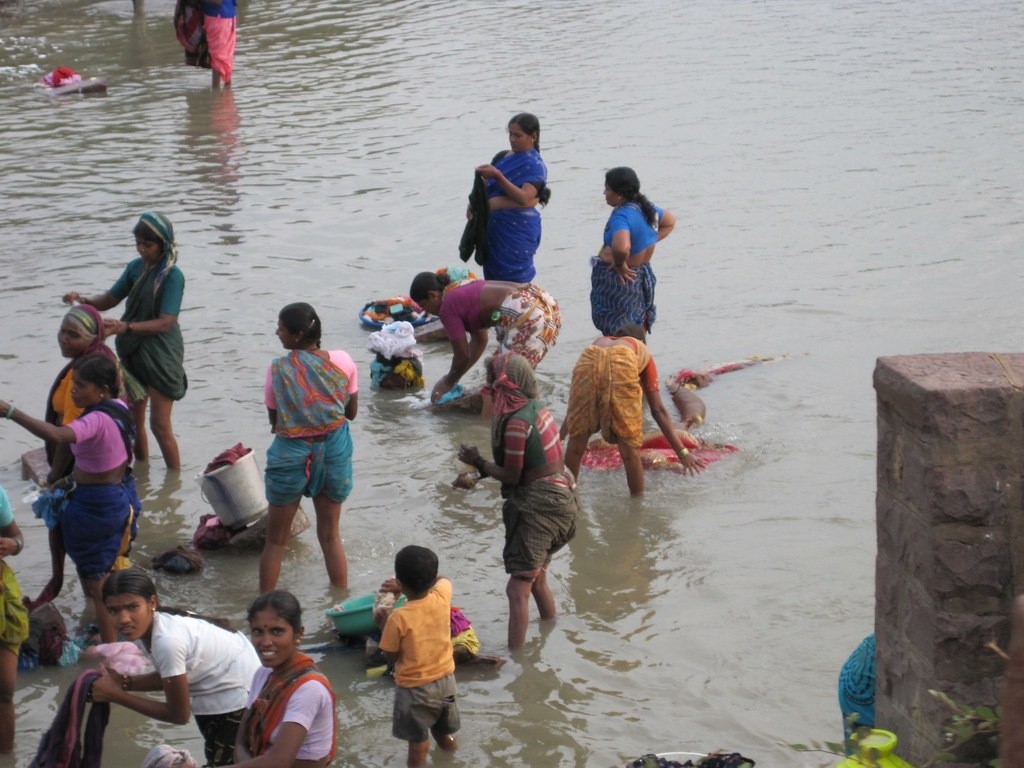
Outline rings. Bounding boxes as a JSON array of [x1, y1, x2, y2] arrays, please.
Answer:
[[436, 392, 438, 394]]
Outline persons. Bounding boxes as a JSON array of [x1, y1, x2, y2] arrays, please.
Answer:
[[449, 354, 578, 649], [174, 1, 238, 93], [563, 321, 707, 497], [257, 302, 358, 593], [374, 544, 461, 768], [0, 210, 335, 768], [459, 112, 551, 282], [589, 167, 675, 335], [409, 271, 561, 419]]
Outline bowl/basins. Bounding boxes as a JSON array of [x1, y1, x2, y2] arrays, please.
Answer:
[[328, 593, 408, 636]]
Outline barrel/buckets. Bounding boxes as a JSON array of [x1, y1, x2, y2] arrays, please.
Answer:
[[194, 447, 272, 530], [626, 751, 745, 768]]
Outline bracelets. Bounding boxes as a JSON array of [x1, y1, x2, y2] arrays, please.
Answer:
[[126, 321, 132, 334], [474, 456, 486, 468], [12, 539, 22, 555], [123, 674, 128, 689], [6, 406, 16, 419], [678, 448, 689, 459]]
